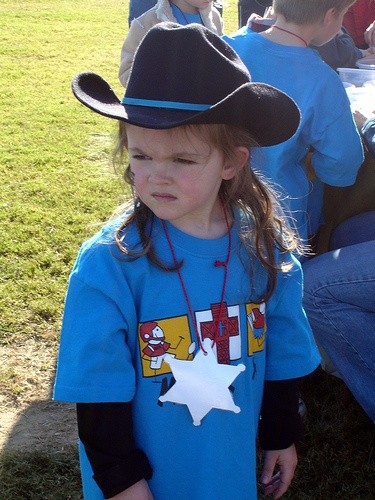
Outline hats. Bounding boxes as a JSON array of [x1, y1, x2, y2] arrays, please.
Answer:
[[72, 22, 301, 147]]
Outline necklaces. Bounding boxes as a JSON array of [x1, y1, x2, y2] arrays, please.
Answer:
[[271, 25, 309, 48], [158, 194, 247, 427], [169, 2, 204, 25]]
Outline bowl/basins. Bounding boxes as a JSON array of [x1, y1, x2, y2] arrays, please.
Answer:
[[335, 57, 375, 115]]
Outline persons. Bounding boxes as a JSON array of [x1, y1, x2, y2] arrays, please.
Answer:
[[218, 0, 366, 418], [116, 0, 225, 89], [128, 0, 224, 30], [240, 1, 375, 76], [53, 23, 321, 500], [300, 111, 375, 420]]
